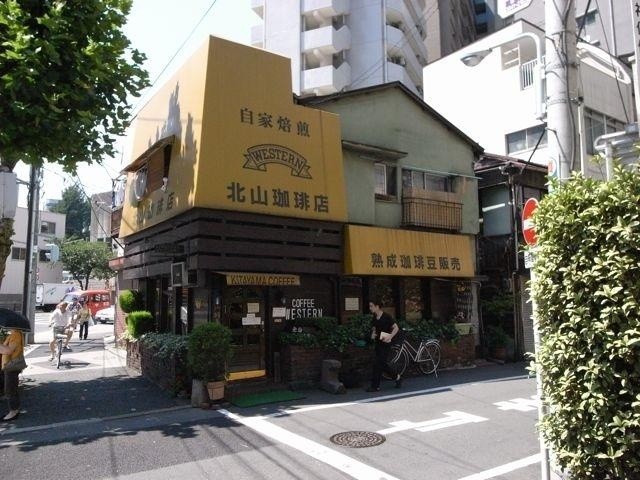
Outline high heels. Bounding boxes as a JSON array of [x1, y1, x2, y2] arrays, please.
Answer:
[[0, 414, 18, 423]]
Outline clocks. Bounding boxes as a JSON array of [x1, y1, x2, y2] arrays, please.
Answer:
[[171, 262, 185, 288]]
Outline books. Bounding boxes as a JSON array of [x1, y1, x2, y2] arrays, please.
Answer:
[[380, 331, 392, 343]]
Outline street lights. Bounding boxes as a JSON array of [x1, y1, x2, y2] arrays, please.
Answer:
[[458, 32, 543, 125]]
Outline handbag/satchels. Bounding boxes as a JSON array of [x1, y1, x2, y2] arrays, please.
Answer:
[[5, 356, 27, 371]]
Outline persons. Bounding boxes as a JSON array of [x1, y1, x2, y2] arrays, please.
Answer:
[[365, 299, 405, 392], [48, 297, 91, 362], [0, 329, 24, 422]]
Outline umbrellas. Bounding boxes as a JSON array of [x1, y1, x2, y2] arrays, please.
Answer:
[[0, 308, 32, 332]]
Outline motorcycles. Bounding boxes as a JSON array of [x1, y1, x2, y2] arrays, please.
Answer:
[[70, 308, 79, 329]]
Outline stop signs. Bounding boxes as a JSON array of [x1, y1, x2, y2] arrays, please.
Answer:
[[520, 199, 539, 248]]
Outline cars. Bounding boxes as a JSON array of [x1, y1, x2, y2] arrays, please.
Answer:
[[94, 306, 114, 323]]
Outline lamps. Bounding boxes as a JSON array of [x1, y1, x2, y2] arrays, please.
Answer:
[[133, 172, 148, 202]]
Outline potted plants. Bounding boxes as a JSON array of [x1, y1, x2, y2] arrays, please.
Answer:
[[486, 325, 513, 360], [187, 322, 234, 402]]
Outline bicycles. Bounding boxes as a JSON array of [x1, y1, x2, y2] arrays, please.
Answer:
[[49, 325, 68, 368], [381, 328, 441, 380]]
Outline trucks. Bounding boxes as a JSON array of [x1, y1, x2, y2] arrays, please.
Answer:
[[34, 282, 82, 313]]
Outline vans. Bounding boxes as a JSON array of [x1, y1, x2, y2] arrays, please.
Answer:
[[59, 289, 111, 318]]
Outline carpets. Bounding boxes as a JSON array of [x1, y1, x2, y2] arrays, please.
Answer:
[[226, 389, 307, 409]]
